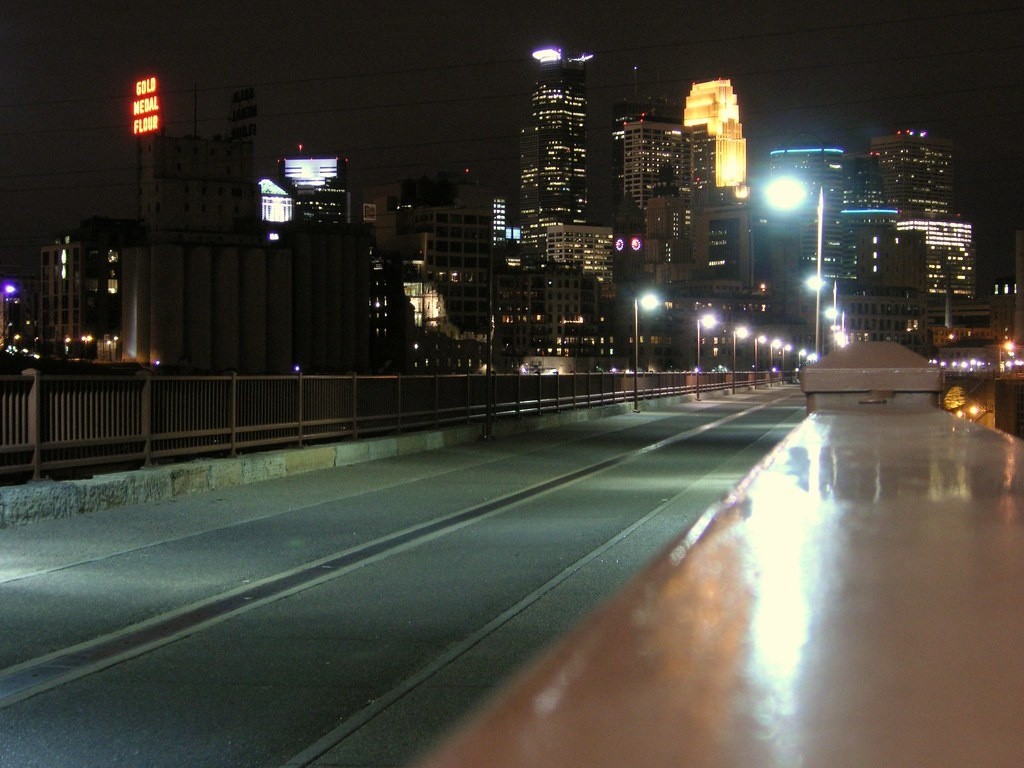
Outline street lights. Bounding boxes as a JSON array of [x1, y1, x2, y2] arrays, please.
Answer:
[[798, 349, 817, 373], [999, 343, 1013, 372], [782, 345, 791, 386], [770, 340, 781, 387], [696, 316, 717, 401], [755, 335, 766, 390], [632, 291, 660, 414], [733, 327, 747, 394], [763, 176, 850, 360]]
